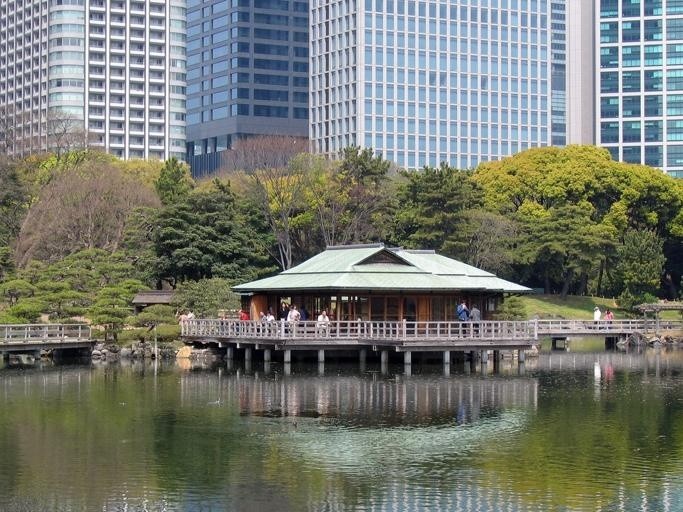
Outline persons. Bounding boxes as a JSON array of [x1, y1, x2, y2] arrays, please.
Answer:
[[239, 310, 249, 332], [469, 304, 481, 338], [317, 311, 330, 337], [593, 306, 601, 329], [604, 309, 614, 329], [458, 300, 470, 338], [188, 310, 196, 319], [259, 302, 309, 334], [177, 312, 187, 321]]
